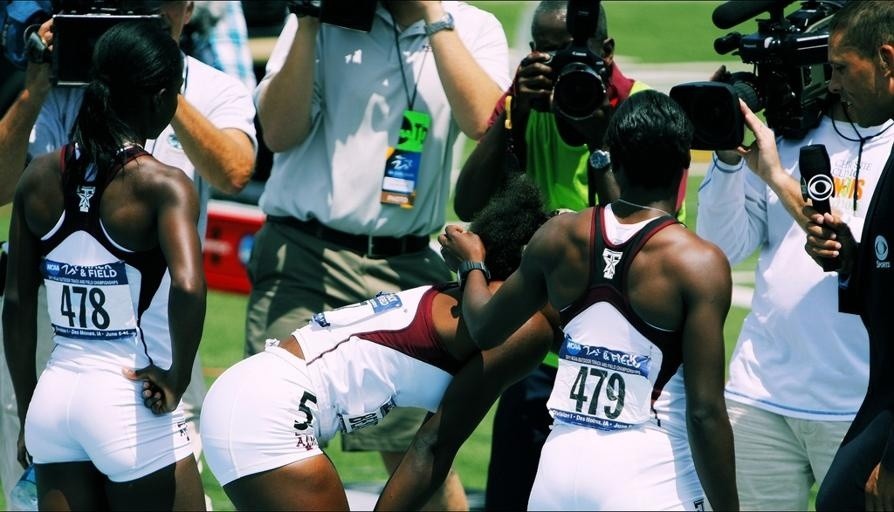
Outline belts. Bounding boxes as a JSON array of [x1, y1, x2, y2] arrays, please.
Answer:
[[267, 213, 430, 259]]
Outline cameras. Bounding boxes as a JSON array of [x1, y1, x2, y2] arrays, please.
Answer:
[[541, 1, 609, 121]]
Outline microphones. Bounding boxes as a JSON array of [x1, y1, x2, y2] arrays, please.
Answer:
[[799, 143, 842, 271]]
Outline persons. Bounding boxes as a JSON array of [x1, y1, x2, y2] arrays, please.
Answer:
[[2, 1, 894, 512]]
